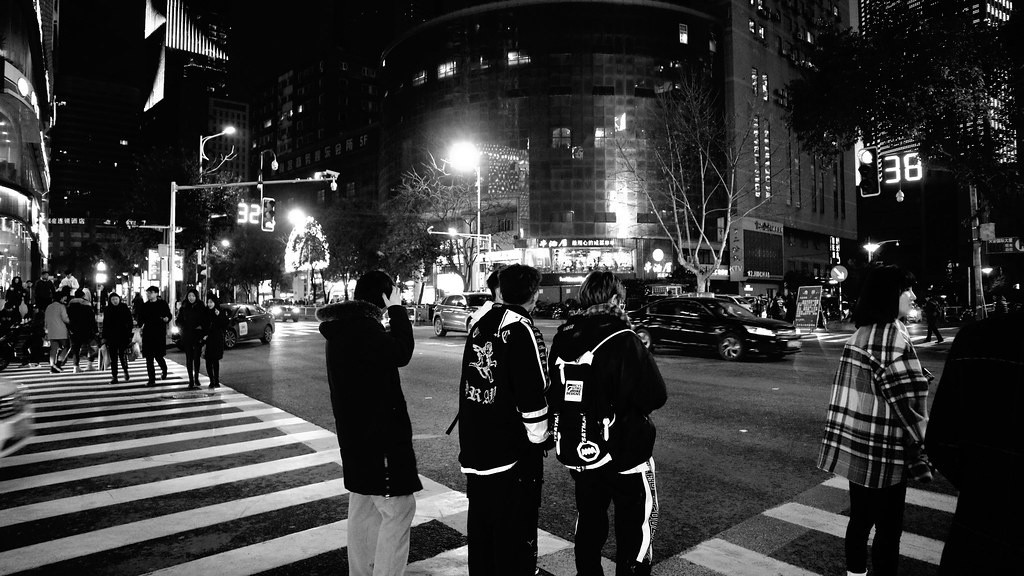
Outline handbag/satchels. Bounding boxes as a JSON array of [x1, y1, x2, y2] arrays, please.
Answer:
[[126, 341, 141, 362]]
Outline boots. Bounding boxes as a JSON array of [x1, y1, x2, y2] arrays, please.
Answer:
[[214, 375, 220, 388], [208, 375, 214, 388]]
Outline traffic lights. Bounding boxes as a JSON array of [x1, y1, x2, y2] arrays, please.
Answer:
[[882, 153, 924, 185], [261, 197, 276, 232], [858, 146, 880, 197], [196, 264, 207, 282], [94, 262, 108, 285], [238, 201, 261, 226]]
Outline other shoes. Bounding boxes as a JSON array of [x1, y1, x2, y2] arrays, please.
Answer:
[[74, 365, 82, 373], [53, 361, 62, 373], [51, 367, 58, 374], [187, 381, 194, 391], [145, 380, 155, 386], [194, 378, 201, 387], [109, 378, 119, 384], [161, 371, 167, 379], [86, 361, 96, 371], [124, 370, 129, 381]]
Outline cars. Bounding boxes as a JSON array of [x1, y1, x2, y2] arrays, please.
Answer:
[[171, 303, 276, 351], [261, 298, 298, 321], [715, 295, 785, 311], [625, 295, 802, 362], [381, 311, 390, 331], [0, 376, 35, 457], [907, 304, 922, 322]]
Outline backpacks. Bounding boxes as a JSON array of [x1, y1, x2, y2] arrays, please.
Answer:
[[548, 328, 615, 471]]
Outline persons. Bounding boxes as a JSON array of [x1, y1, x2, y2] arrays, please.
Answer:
[[101, 292, 133, 385], [0, 270, 99, 373], [135, 286, 172, 386], [132, 292, 144, 328], [817, 266, 937, 576], [459, 263, 553, 576], [756, 289, 831, 323], [923, 294, 944, 343], [549, 271, 668, 576], [316, 271, 423, 576], [176, 290, 229, 387], [923, 309, 1024, 576]]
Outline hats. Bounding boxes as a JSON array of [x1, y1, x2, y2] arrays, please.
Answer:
[[187, 289, 199, 300]]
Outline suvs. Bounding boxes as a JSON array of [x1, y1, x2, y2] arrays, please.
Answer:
[[432, 292, 493, 337]]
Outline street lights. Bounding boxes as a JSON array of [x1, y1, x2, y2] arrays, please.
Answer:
[[449, 142, 480, 291], [198, 125, 237, 301], [869, 240, 900, 262]]
